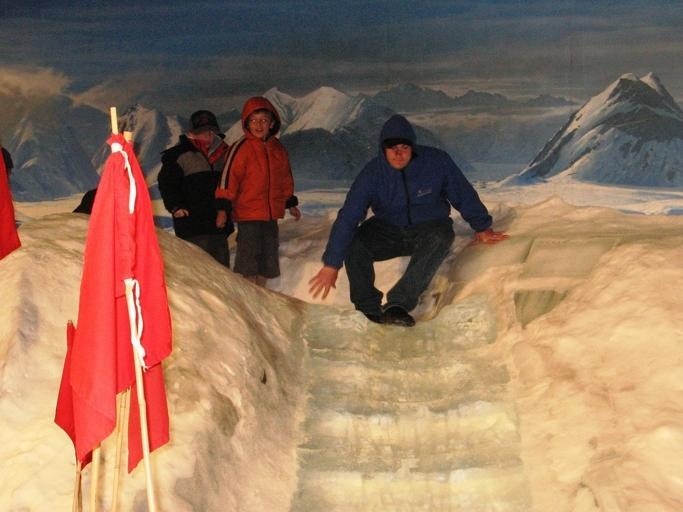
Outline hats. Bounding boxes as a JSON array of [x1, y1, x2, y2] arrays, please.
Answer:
[[190, 111, 219, 135]]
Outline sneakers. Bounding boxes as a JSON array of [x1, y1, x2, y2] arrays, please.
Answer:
[[366, 306, 416, 327]]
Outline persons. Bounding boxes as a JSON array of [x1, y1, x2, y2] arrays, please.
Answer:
[[71, 188, 99, 214], [308, 114, 510, 325], [214, 96, 300, 288], [156, 110, 234, 273]]
